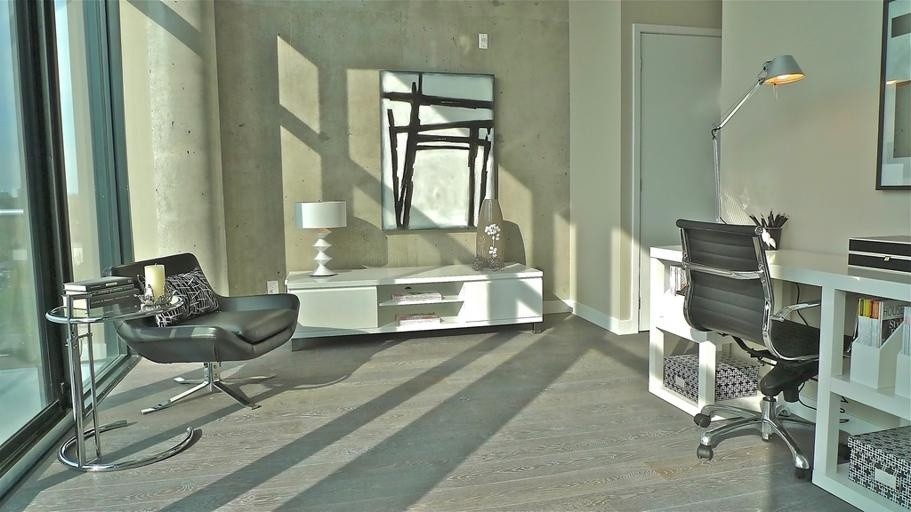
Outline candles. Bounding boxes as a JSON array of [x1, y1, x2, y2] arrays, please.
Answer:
[[142, 261, 166, 302]]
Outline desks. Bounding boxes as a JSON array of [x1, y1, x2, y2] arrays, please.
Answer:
[[645, 245, 910, 511]]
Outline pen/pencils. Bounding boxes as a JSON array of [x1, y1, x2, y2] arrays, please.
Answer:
[[749, 210, 789, 227]]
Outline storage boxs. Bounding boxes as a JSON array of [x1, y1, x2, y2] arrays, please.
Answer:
[[664, 353, 759, 405], [844, 425, 910, 512]]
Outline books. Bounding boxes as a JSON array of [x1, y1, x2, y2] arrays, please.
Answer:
[[857, 294, 910, 345], [64, 284, 134, 297], [60, 299, 140, 318], [389, 290, 442, 304], [58, 288, 139, 312], [398, 312, 442, 327], [62, 275, 134, 293]]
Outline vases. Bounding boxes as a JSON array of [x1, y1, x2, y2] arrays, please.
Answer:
[[476, 200, 505, 269]]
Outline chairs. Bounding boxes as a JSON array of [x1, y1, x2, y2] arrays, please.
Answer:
[[103, 253, 300, 414], [675, 219, 821, 480]]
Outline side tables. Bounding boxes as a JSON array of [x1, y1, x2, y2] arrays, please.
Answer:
[[44, 294, 195, 474]]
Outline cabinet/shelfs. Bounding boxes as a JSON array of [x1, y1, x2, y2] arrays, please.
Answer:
[[286, 262, 545, 353]]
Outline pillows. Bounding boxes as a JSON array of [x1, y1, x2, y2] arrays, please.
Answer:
[[138, 264, 219, 327]]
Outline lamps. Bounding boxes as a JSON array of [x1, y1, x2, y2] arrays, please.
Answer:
[[711, 53, 806, 222], [296, 201, 350, 276]]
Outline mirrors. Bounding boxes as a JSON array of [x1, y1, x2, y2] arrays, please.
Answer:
[[876, 0, 911, 189]]
